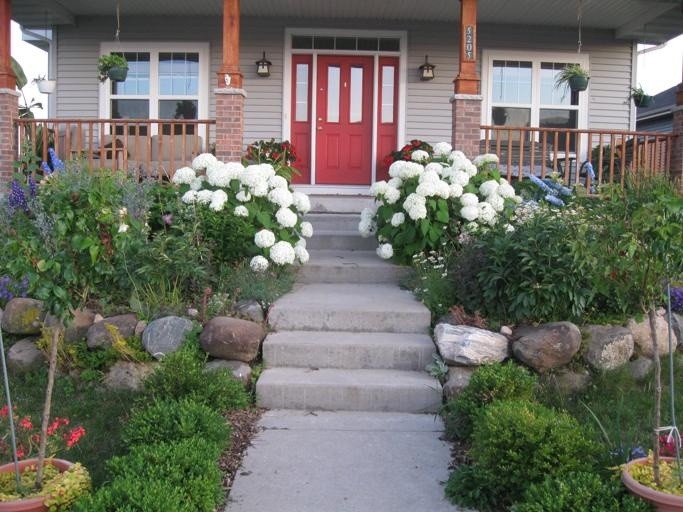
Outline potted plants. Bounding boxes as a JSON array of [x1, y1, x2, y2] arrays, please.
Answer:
[[96, 54, 129, 83], [572, 193, 683, 512], [625, 83, 656, 112], [554, 61, 590, 103], [0, 173, 143, 512]]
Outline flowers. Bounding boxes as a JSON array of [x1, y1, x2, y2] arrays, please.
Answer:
[[0, 403, 87, 491]]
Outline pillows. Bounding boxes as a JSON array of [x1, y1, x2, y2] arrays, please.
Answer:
[[97, 138, 131, 160]]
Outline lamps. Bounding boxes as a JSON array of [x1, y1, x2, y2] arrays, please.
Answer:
[[255, 51, 272, 78], [418, 53, 436, 81]]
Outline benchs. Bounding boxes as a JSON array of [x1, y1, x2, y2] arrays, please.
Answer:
[[479, 139, 553, 178]]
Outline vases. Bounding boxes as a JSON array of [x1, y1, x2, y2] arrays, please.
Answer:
[[35, 79, 57, 95]]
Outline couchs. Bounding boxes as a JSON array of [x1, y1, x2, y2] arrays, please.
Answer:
[[83, 134, 202, 177]]
